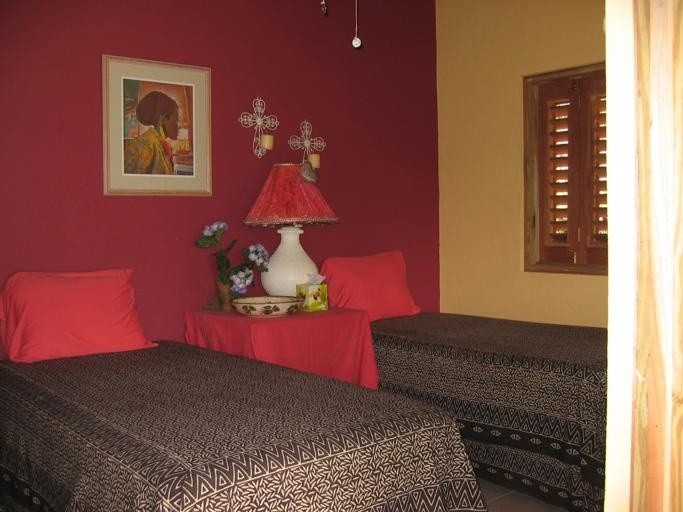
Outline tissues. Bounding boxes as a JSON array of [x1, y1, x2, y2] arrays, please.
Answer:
[[295, 272, 329, 313]]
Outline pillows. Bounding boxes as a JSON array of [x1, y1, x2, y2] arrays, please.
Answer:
[[0, 268, 159, 363], [321, 251, 422, 322]]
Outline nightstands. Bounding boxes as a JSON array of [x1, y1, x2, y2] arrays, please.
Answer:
[[185, 308, 380, 390]]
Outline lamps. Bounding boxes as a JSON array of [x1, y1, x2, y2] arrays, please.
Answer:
[[243, 163, 339, 297]]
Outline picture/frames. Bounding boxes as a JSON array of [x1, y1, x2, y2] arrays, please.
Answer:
[[102, 54, 212, 196]]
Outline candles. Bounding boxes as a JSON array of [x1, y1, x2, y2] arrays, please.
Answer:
[[261, 135, 274, 150], [308, 151, 320, 168]]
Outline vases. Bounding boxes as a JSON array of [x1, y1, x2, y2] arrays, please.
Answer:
[[217, 282, 235, 310]]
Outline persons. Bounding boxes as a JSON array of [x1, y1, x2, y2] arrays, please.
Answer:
[[124, 92, 179, 175]]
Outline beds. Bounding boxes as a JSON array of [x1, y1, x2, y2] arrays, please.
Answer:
[[0, 340, 489, 512], [370, 311, 607, 511]]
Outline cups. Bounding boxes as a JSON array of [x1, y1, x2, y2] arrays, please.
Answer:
[[566, 223, 588, 266]]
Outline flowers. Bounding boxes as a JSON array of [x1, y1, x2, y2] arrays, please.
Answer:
[[197, 221, 270, 294]]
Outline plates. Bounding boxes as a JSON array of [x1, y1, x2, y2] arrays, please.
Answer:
[[231, 294, 304, 320]]
